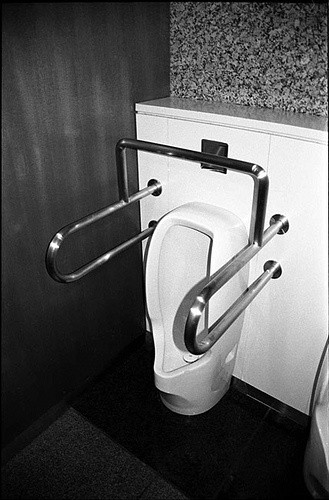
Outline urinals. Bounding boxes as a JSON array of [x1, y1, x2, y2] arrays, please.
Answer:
[[141, 201, 249, 415]]
